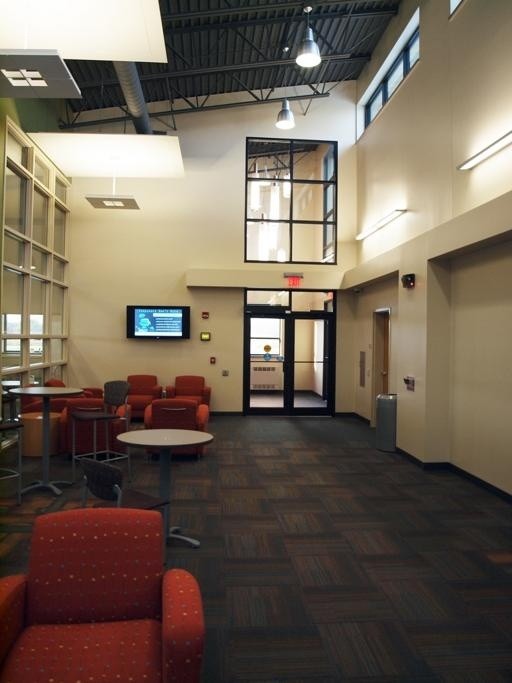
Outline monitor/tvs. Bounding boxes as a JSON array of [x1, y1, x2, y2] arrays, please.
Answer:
[[126, 305, 191, 341]]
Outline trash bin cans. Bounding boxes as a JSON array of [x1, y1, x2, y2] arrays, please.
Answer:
[[375, 393, 397, 452]]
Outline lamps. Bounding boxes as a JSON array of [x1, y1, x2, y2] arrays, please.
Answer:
[[456, 130, 512, 171], [84, 178, 140, 210], [355, 210, 406, 241], [0, 48, 84, 99], [295, 6, 322, 68], [247, 154, 291, 260], [273, 47, 295, 130]]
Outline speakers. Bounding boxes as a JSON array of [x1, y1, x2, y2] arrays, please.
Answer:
[[400, 273, 415, 289]]
